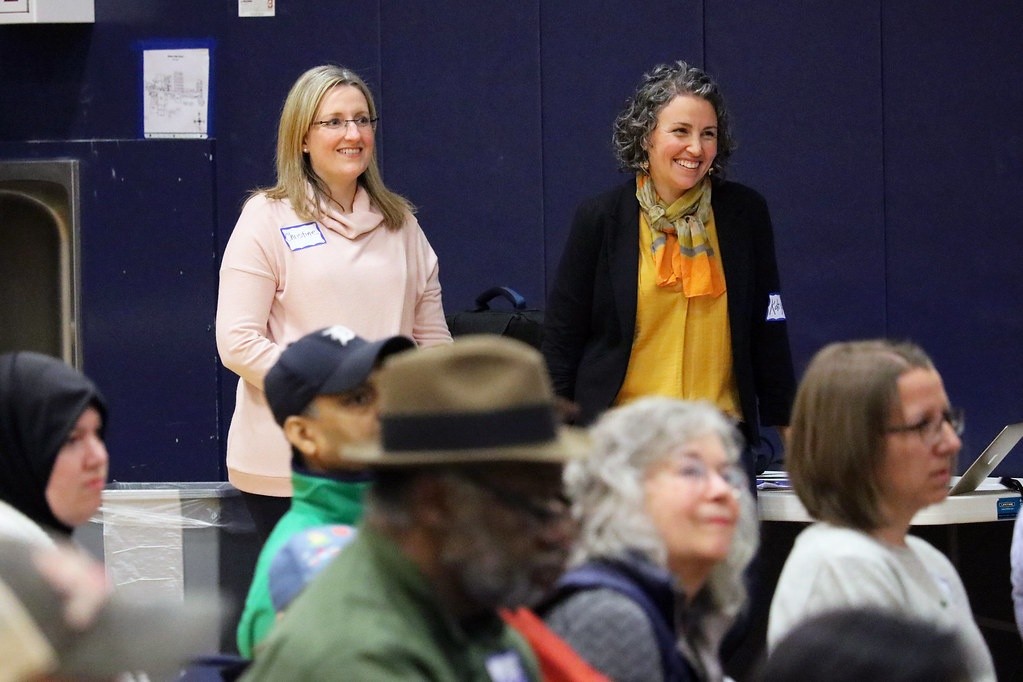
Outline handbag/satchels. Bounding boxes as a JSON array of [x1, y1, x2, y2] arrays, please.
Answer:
[[445, 287, 548, 350]]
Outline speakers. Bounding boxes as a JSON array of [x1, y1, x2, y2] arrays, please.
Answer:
[[0, 158, 83, 379]]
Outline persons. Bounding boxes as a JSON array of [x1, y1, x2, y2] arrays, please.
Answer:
[[540, 62, 798, 449], [239, 337, 619, 682], [536, 399, 760, 682], [0, 352, 150, 682], [215, 65, 456, 543], [767, 340, 995, 682], [767, 611, 970, 682], [238, 325, 420, 658]]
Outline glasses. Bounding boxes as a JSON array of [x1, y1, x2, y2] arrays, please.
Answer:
[[649, 455, 748, 500], [307, 112, 379, 136], [446, 464, 587, 523], [885, 403, 965, 445]]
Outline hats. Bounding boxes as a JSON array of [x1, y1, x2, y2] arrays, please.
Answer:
[[0, 351, 108, 536], [336, 335, 594, 464], [263, 325, 416, 429]]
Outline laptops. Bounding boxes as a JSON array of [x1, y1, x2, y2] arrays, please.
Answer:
[[946, 423, 1023, 496]]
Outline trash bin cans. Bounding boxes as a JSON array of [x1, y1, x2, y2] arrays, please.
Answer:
[[72, 482, 261, 681]]
